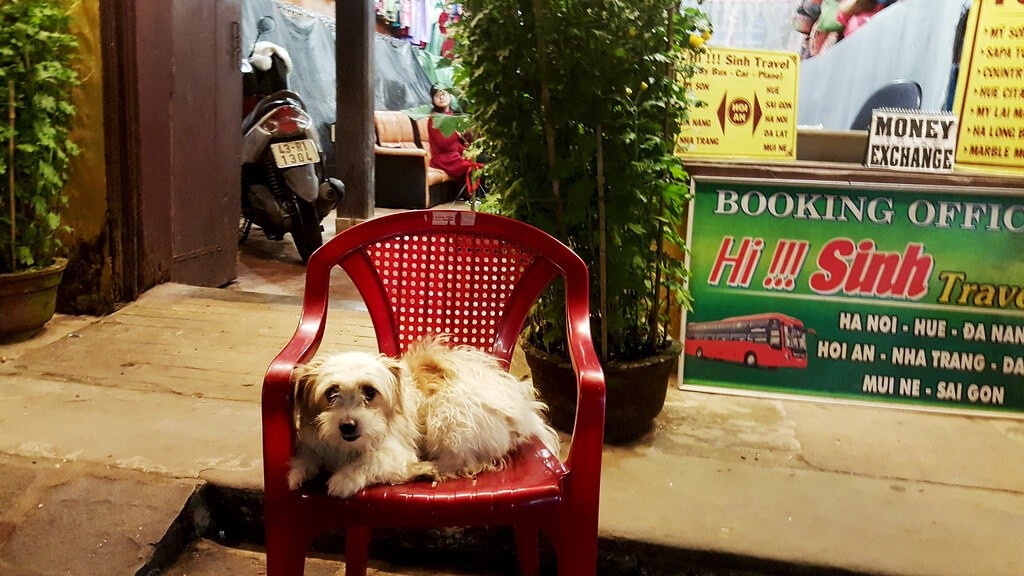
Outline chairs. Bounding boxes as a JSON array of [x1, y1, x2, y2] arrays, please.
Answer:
[[260, 211, 608, 576]]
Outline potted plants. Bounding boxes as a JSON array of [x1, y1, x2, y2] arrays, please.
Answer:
[[0, 0, 81, 346], [433, 0, 708, 439]]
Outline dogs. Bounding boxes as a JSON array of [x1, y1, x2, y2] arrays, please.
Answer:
[[289, 330, 560, 501]]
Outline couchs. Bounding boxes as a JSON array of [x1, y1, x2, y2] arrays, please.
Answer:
[[328, 109, 487, 208]]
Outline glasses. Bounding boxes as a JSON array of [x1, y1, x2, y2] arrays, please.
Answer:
[[433, 91, 449, 99]]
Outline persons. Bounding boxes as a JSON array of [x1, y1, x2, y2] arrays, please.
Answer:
[[427, 81, 486, 203]]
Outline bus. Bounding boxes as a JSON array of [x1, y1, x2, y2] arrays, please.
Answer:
[[685, 312, 819, 371]]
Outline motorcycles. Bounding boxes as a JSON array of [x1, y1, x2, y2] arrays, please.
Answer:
[[238, 15, 347, 266]]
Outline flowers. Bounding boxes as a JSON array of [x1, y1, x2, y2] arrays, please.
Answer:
[[430, 13, 460, 61]]
